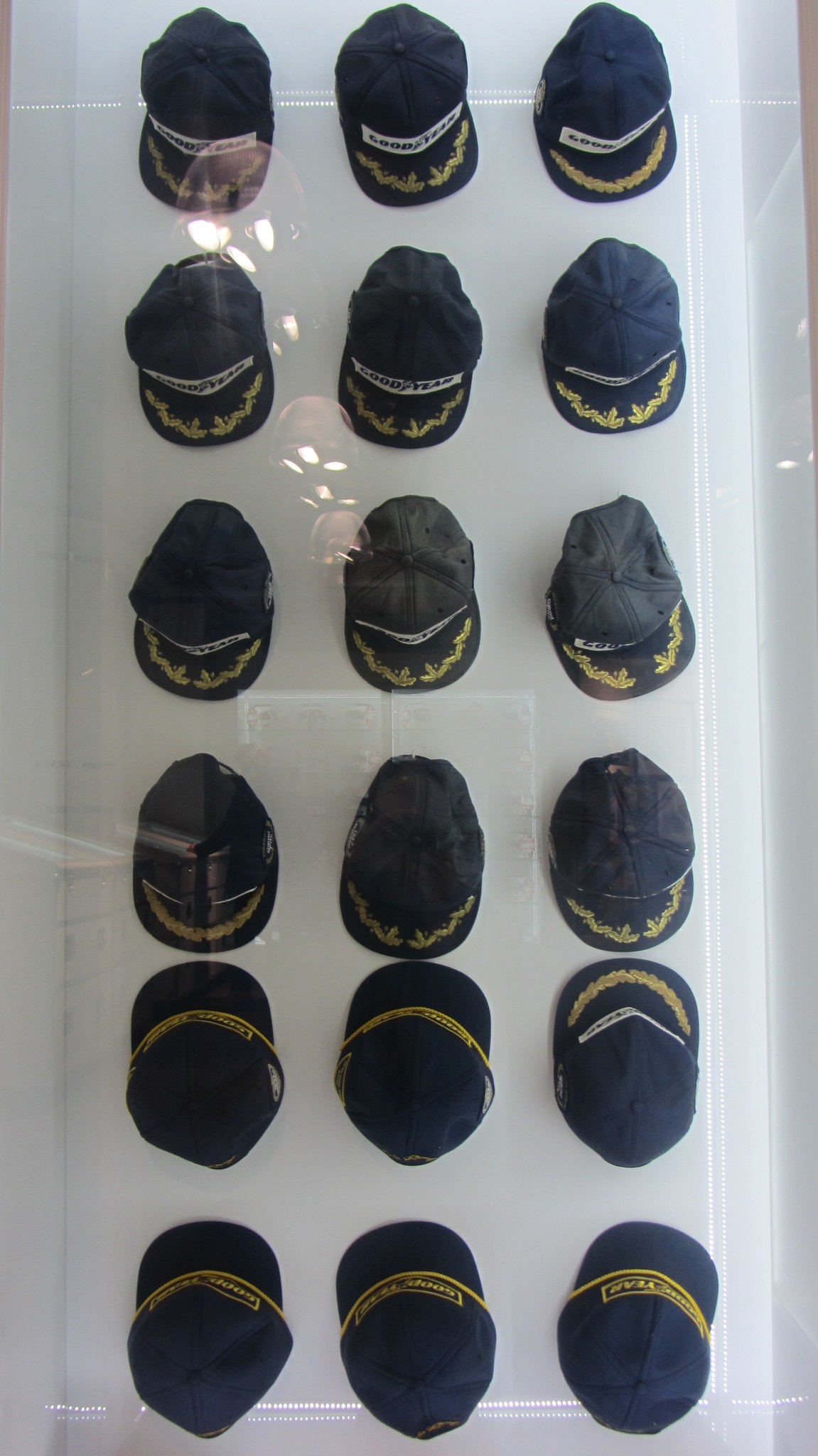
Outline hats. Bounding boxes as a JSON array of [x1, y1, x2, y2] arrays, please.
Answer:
[[540, 234, 687, 433], [344, 495, 480, 695], [553, 958, 701, 1169], [338, 246, 483, 450], [547, 495, 696, 701], [129, 499, 275, 702], [125, 252, 274, 446], [139, 6, 275, 212], [335, 961, 495, 1167], [532, 4, 678, 205], [548, 748, 698, 954], [339, 754, 484, 960], [335, 1221, 496, 1440], [557, 1221, 719, 1434], [127, 1221, 293, 1440], [334, 4, 478, 206], [126, 962, 284, 1170], [132, 754, 278, 954]]
